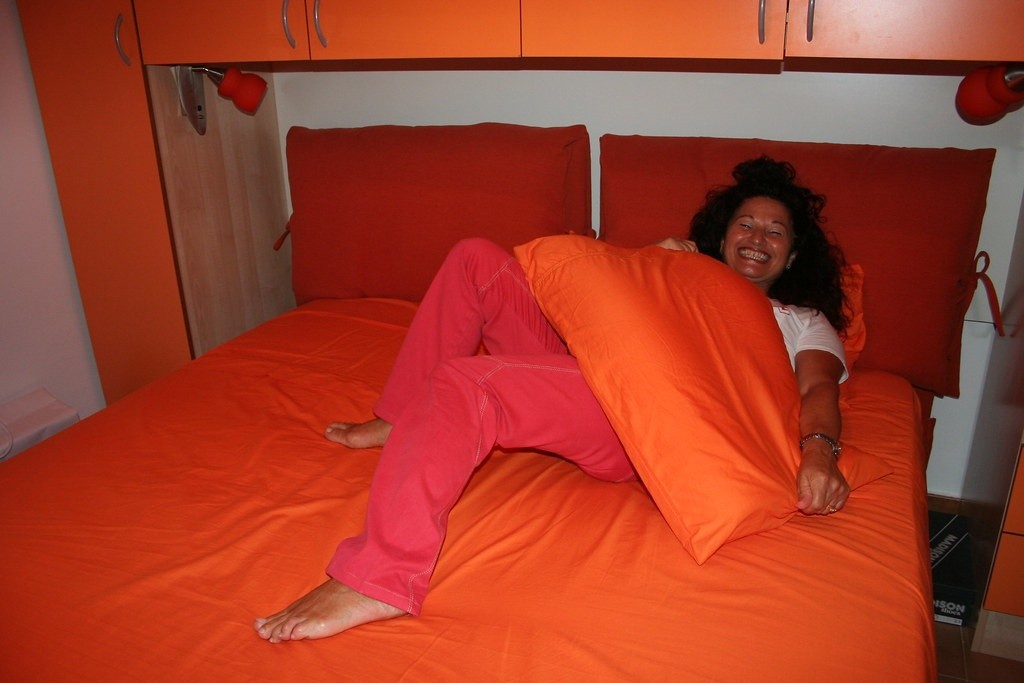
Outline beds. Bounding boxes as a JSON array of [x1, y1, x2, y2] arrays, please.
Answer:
[[0, 298, 942, 683]]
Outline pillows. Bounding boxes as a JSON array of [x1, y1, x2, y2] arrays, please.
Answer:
[[285, 121, 597, 308], [513, 238, 894, 566], [598, 133, 998, 400]]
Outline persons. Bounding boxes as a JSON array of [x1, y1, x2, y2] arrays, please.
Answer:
[[254, 154, 850, 643]]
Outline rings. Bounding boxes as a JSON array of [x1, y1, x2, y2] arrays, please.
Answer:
[[827, 506, 836, 512]]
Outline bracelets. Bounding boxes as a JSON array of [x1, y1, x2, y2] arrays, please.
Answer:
[[800, 432, 840, 455]]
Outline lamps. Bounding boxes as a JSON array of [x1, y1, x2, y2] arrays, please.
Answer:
[[955, 67, 1024, 126], [176, 66, 269, 116]]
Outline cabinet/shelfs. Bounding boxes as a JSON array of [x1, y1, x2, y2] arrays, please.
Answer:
[[16, 0, 297, 407], [521, 0, 1024, 78], [133, 0, 522, 73]]
